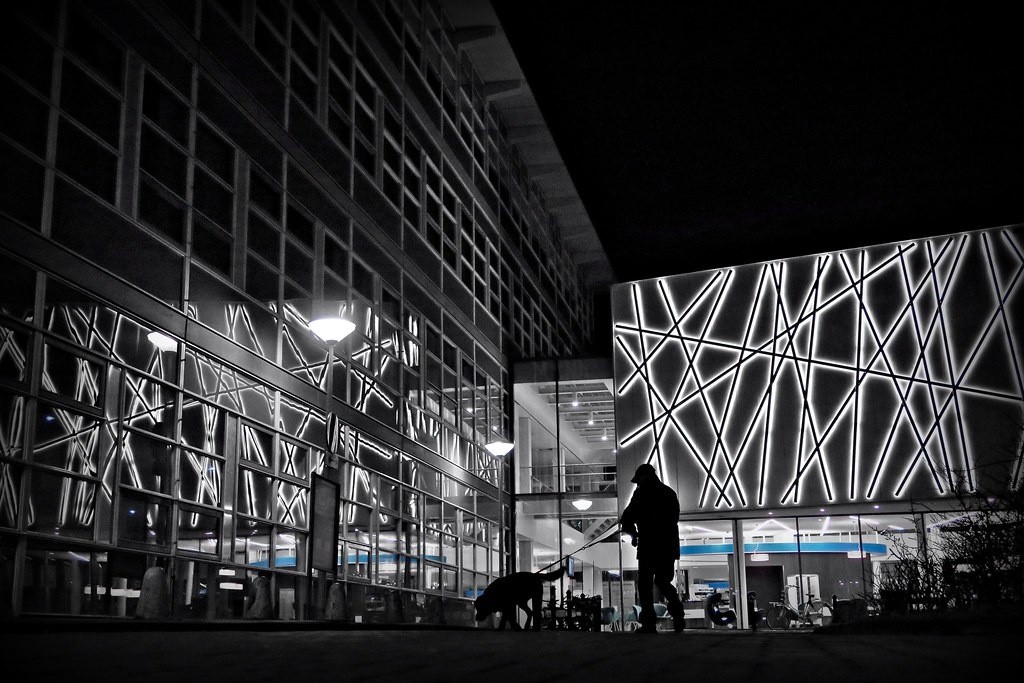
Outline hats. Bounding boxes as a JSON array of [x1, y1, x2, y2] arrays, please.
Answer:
[[631, 464, 654, 483]]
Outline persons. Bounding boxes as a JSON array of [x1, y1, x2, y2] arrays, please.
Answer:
[[619, 463, 686, 633]]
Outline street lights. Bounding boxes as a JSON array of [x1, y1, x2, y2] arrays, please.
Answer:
[[484, 440, 516, 579], [304, 316, 356, 619]]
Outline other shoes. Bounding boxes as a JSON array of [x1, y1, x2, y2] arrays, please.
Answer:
[[635, 625, 657, 634], [673, 611, 686, 633]]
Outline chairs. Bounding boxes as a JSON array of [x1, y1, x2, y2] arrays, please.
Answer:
[[599, 603, 672, 631]]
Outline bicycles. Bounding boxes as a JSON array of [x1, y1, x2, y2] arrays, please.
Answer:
[[766, 585, 834, 632]]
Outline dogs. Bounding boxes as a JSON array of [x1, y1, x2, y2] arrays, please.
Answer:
[[472, 565, 569, 631]]
